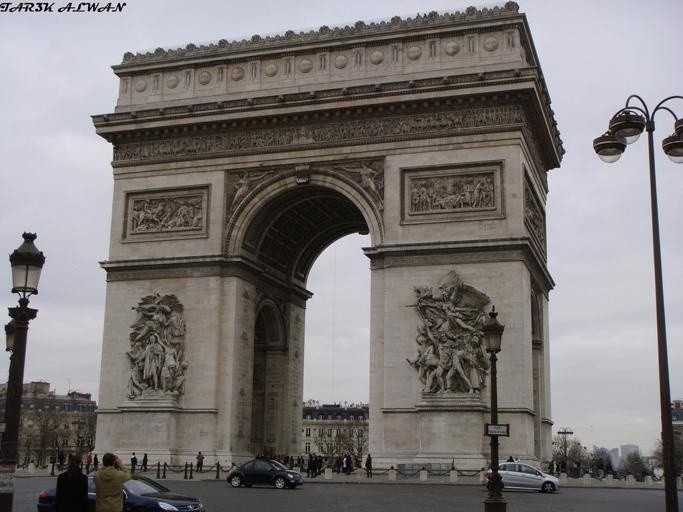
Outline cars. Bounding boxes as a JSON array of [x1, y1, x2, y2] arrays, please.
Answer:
[[37, 473, 206, 511], [482, 462, 561, 494], [228, 458, 304, 490]]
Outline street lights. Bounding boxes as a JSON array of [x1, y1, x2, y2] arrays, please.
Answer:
[[594, 90, 683, 512], [480, 303, 511, 511], [0, 230, 45, 511]]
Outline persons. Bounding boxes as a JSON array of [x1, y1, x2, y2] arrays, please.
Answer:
[[547, 457, 617, 480], [513, 456, 521, 472], [112, 102, 523, 163], [409, 176, 494, 211], [92, 452, 131, 512], [125, 295, 190, 399], [195, 451, 204, 472], [332, 161, 383, 212], [506, 455, 514, 470], [253, 451, 373, 479], [55, 454, 88, 511], [56, 449, 147, 476], [227, 169, 276, 212], [129, 196, 203, 231], [406, 278, 491, 394]]
